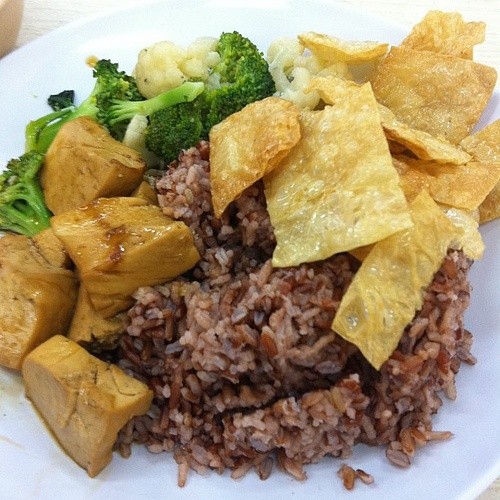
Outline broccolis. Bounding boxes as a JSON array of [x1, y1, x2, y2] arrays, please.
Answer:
[[0, 30, 277, 235]]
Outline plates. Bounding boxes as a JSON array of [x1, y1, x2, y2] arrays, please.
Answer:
[[0, 0, 499, 500]]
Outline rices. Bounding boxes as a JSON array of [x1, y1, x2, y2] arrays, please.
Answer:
[[102, 140, 477, 492]]
